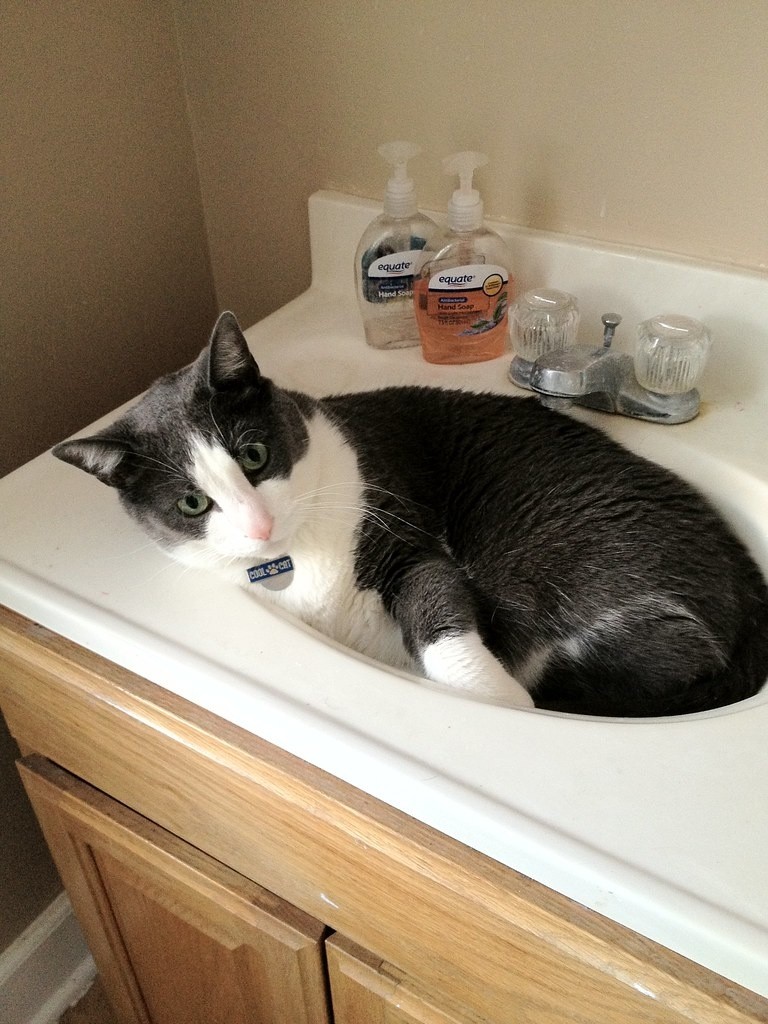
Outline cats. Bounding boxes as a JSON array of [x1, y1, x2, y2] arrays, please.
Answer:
[[50, 311, 768, 716]]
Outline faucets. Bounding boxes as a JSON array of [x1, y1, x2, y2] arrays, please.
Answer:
[[529, 341, 635, 415]]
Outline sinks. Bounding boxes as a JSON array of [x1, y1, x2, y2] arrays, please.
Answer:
[[219, 379, 768, 723]]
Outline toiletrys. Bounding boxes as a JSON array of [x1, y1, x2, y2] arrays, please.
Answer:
[[351, 141, 521, 365]]
